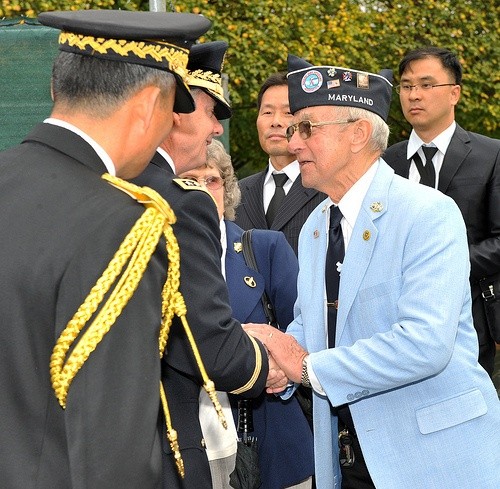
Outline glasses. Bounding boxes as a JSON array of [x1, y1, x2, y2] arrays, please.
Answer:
[[182, 175, 225, 190], [394, 81, 458, 95], [286, 118, 357, 143]]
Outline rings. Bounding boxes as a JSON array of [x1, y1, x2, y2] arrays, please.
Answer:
[[269, 332, 273, 337]]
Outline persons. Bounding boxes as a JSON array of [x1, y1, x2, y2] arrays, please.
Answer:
[[126, 40, 280, 489], [240, 54, 500, 489], [177, 139, 315, 489], [380, 48, 500, 377], [235, 69, 329, 257], [0, 10, 227, 489]]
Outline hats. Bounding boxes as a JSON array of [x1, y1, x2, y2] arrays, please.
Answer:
[[38, 9, 212, 114], [186, 40, 233, 120], [284, 53, 396, 122]]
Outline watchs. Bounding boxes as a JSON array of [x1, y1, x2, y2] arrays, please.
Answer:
[[301, 355, 312, 387]]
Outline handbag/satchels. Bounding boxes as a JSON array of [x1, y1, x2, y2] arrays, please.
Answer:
[[480, 274, 500, 344], [301, 397, 376, 489]]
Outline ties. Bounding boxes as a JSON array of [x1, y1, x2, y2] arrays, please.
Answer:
[[417, 146, 440, 188], [265, 173, 289, 230], [325, 205, 346, 349]]
